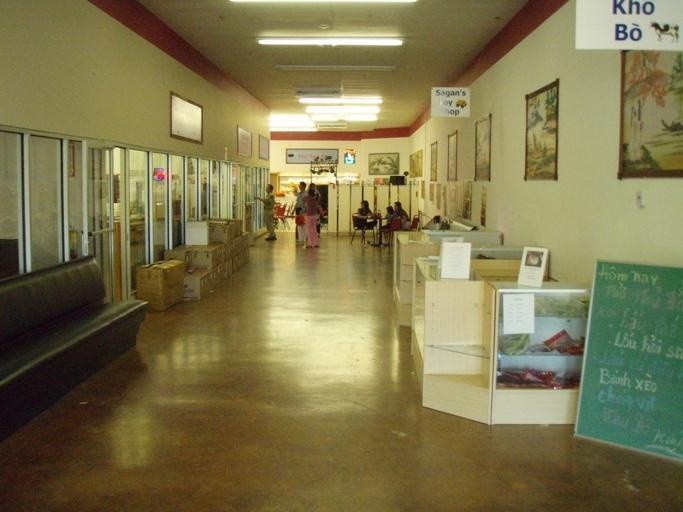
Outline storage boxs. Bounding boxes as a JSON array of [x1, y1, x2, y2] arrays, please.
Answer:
[[135, 217, 250, 312]]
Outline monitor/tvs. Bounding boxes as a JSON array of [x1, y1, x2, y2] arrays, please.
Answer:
[[389, 176, 405, 185]]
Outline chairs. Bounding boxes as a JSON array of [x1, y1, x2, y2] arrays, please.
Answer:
[[350, 213, 420, 247]]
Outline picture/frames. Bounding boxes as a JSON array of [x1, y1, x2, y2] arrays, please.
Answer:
[[168, 89, 204, 145], [408, 50, 681, 182], [236, 124, 252, 159], [258, 134, 269, 161], [286, 148, 339, 165], [368, 152, 400, 176]]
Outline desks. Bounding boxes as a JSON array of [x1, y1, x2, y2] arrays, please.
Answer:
[[110, 213, 145, 303]]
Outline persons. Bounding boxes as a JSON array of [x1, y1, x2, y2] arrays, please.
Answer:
[[290, 180, 328, 247], [355, 198, 409, 242], [254, 184, 279, 241]]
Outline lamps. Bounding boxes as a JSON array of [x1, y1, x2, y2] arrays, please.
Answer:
[[255, 34, 408, 50], [273, 63, 399, 74]]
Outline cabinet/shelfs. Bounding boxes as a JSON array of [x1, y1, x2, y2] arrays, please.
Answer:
[[390, 230, 504, 328], [410, 255, 594, 426]]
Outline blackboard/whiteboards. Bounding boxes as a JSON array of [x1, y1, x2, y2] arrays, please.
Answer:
[[574, 259, 683, 466]]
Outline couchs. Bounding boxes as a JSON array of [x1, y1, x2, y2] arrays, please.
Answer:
[[0, 256, 147, 443]]
